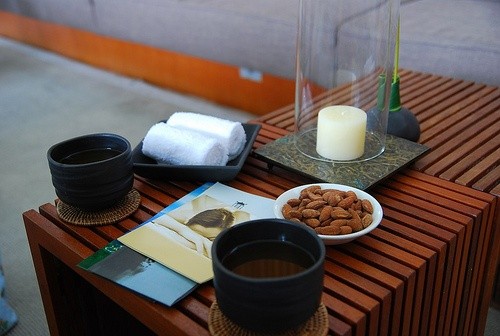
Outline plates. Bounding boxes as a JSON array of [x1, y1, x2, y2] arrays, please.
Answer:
[[124, 120, 262, 180]]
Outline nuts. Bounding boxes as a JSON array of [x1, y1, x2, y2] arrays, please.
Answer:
[[282, 186, 373, 236]]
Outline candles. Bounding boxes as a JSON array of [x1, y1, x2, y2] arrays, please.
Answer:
[[316, 105, 367, 160]]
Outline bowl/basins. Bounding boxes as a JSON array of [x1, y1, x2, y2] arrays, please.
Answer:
[[274, 183, 383, 245]]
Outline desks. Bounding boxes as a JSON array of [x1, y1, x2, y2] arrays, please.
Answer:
[[23, 67, 500, 336]]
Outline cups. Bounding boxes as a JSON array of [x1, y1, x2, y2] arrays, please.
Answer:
[[211, 217, 326, 331], [47, 133, 135, 213], [294, 0, 399, 164]]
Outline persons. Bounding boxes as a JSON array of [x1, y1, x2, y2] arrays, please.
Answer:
[[152, 207, 236, 261]]
